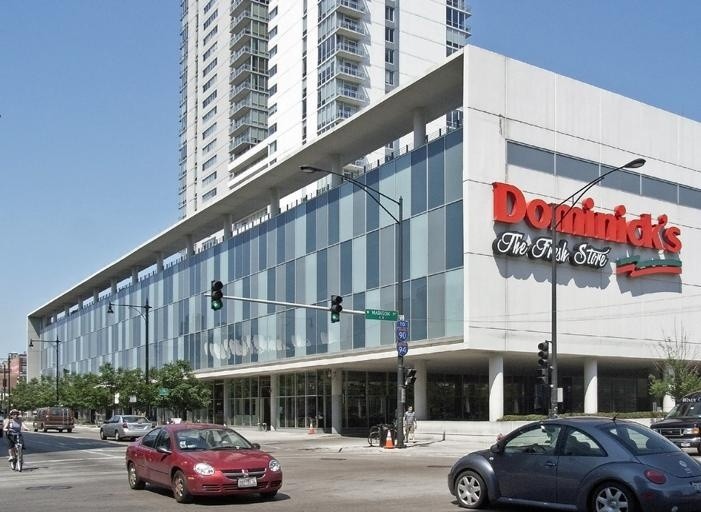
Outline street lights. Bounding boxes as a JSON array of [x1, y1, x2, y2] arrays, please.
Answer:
[[551, 155, 647, 418], [299, 163, 407, 451], [0, 357, 9, 416], [28, 336, 62, 404], [106, 298, 153, 418]]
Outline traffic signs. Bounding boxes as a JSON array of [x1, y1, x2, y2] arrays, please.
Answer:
[[366, 308, 399, 322]]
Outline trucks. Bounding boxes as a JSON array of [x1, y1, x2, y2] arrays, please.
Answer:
[[649, 386, 701, 459]]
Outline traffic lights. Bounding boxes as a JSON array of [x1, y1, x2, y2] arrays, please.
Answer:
[[537, 342, 548, 385], [403, 368, 417, 387], [329, 295, 343, 322], [210, 280, 224, 310]]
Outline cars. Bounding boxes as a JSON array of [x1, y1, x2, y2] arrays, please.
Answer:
[[126, 423, 284, 504], [100, 415, 155, 441], [446, 418, 701, 512]]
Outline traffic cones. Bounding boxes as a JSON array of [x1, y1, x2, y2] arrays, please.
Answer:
[[385, 428, 396, 449]]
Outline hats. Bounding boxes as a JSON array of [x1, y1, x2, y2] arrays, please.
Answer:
[[10, 408, 20, 415]]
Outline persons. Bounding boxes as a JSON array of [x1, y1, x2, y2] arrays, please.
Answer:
[[2, 409, 30, 461], [403, 404, 417, 443]]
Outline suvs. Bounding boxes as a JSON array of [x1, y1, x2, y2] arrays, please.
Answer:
[[33, 405, 76, 435], [0, 409, 6, 439]]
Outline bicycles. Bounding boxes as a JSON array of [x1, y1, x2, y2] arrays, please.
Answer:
[[6, 428, 31, 475], [366, 423, 396, 448]]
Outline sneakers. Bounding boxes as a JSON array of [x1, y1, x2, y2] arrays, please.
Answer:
[[9, 456, 14, 461]]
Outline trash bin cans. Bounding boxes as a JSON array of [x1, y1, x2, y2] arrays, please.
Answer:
[[380, 424, 395, 447]]
